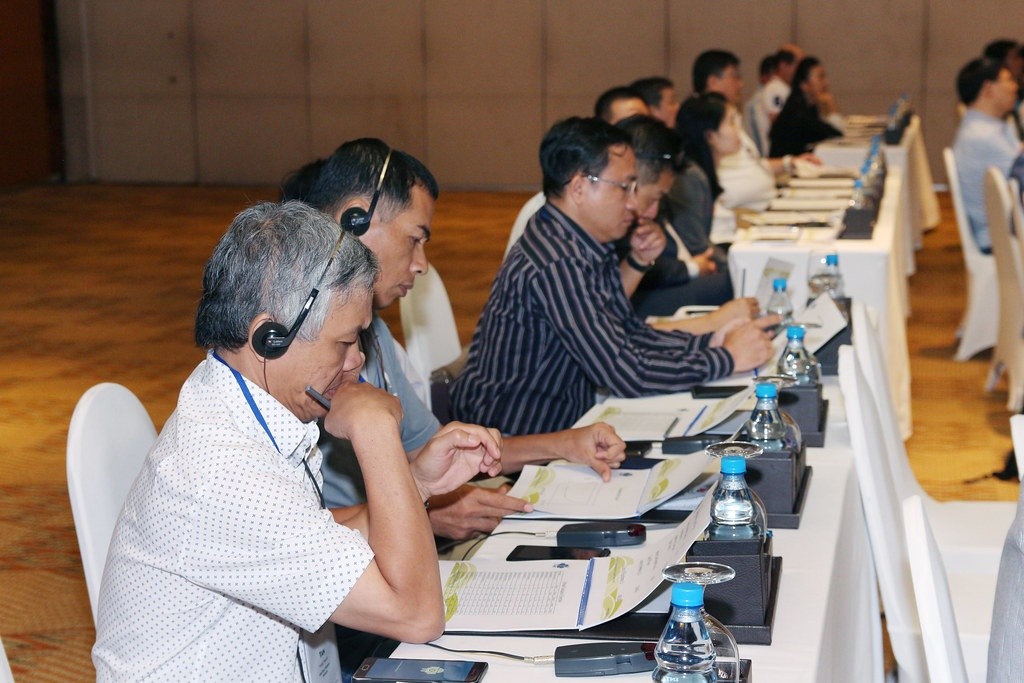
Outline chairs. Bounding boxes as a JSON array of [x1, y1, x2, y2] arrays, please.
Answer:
[[63, 382, 162, 637], [396, 258, 463, 407], [839, 104, 1024, 683]]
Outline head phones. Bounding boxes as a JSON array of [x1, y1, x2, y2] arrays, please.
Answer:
[[253, 230, 345, 360], [341, 146, 394, 236]]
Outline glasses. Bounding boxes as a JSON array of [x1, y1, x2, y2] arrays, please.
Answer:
[[569, 168, 637, 196], [637, 151, 686, 163]]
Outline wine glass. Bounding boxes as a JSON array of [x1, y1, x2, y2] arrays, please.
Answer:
[[778, 322, 823, 387], [704, 441, 768, 543], [747, 374, 802, 455], [652, 562, 741, 683]]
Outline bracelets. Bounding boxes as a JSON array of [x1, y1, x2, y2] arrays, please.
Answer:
[[424, 499, 430, 518], [782, 154, 792, 173]]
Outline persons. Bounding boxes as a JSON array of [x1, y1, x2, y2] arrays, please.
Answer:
[[955, 37, 1024, 255], [91, 199, 503, 683], [281, 43, 847, 494], [297, 137, 627, 540]]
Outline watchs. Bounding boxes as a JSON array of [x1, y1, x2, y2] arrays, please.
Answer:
[[626, 250, 655, 273]]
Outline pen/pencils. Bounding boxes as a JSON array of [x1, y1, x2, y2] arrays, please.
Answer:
[[741, 269, 746, 297], [305, 386, 331, 410]]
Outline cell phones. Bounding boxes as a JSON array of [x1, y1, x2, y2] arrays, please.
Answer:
[[617, 454, 666, 470], [351, 656, 488, 683], [506, 545, 609, 562], [691, 385, 748, 399]]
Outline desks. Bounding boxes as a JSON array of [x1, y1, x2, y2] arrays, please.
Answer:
[[373, 297, 904, 683], [725, 168, 915, 441], [798, 111, 942, 279]]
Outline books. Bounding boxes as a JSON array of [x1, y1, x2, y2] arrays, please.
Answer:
[[709, 292, 846, 386], [573, 381, 760, 439], [500, 417, 749, 520], [439, 479, 720, 632]]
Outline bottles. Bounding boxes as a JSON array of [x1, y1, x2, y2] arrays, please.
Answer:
[[767, 278, 793, 325], [777, 326, 817, 387], [749, 384, 787, 452], [810, 254, 841, 299], [708, 456, 761, 541], [850, 134, 882, 210], [655, 582, 719, 683]]
